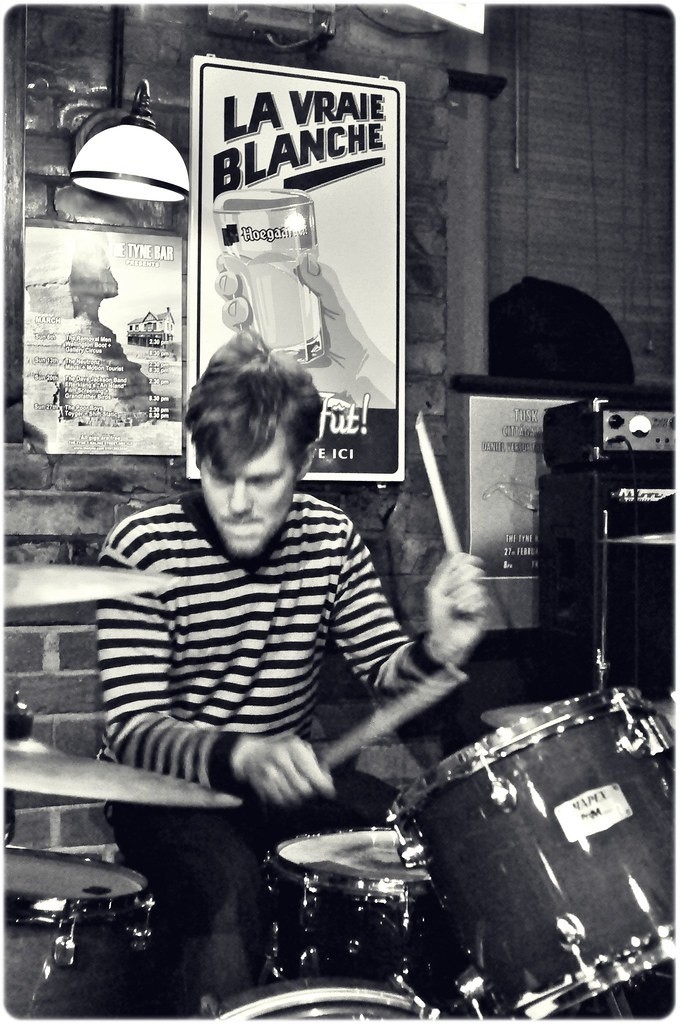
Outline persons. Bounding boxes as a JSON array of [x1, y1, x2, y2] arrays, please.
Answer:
[[95, 357, 490, 1017]]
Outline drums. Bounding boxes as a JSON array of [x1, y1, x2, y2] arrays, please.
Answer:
[[259, 829, 467, 1005], [195, 975, 448, 1019], [4, 847, 158, 1018], [390, 684, 675, 1019]]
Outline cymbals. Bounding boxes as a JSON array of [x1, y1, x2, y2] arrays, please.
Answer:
[[480, 700, 673, 735], [6, 703, 243, 808], [3, 562, 178, 604]]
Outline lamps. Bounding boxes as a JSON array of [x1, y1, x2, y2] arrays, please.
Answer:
[[71, 79, 191, 202]]
